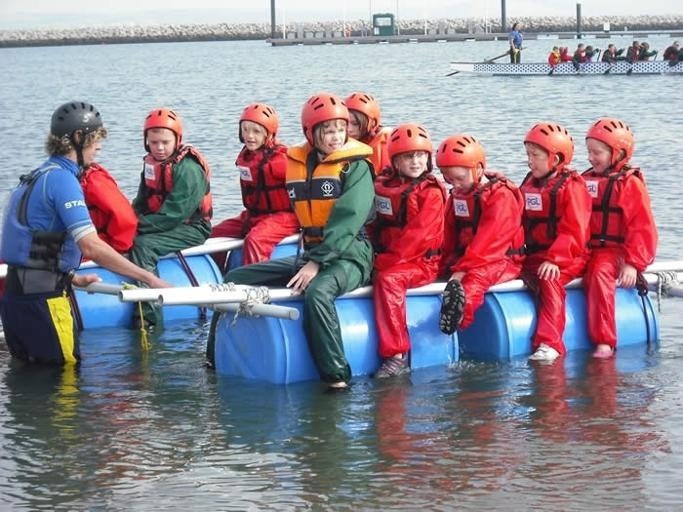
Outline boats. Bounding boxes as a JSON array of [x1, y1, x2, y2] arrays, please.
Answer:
[[0, 234, 305, 335], [448, 60, 682, 77], [205, 249, 662, 387], [210, 334, 654, 449]]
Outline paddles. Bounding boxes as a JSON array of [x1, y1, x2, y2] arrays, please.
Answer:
[[446, 46, 529, 77], [626, 61, 637, 75], [549, 57, 561, 75], [604, 63, 615, 75]]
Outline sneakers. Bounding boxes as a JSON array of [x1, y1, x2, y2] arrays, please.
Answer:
[[592, 349, 615, 359], [372, 353, 411, 378], [529, 344, 559, 360], [440, 280, 465, 335]]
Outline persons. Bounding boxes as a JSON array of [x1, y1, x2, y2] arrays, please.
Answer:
[[507, 23, 682, 65], [128, 109, 215, 326], [210, 102, 300, 266], [375, 127, 450, 379], [82, 159, 137, 261], [1, 103, 173, 368], [580, 119, 658, 357], [435, 137, 526, 333], [520, 122, 595, 361], [206, 93, 373, 395], [343, 93, 393, 172]]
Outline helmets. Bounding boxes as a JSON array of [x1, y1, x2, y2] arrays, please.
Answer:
[[301, 95, 349, 146], [143, 108, 182, 151], [436, 134, 487, 171], [523, 122, 574, 171], [585, 116, 632, 174], [239, 104, 279, 142], [51, 100, 103, 139], [387, 123, 432, 173], [346, 93, 380, 129]]
